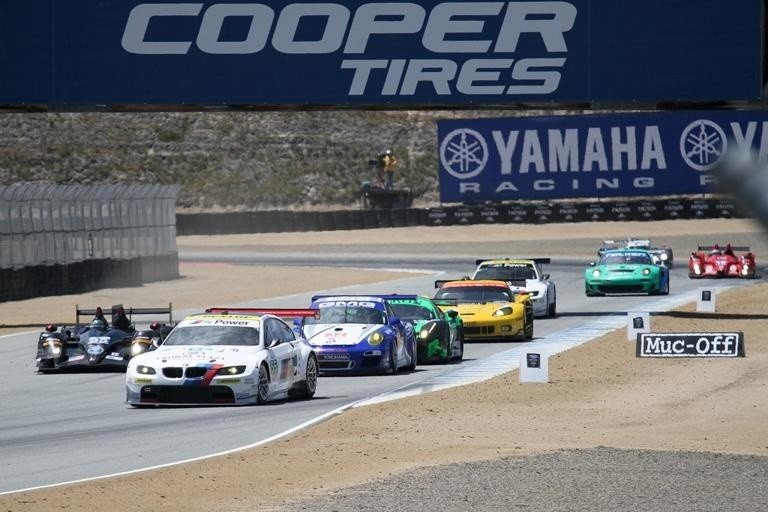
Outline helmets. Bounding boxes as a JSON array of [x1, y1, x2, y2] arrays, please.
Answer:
[[91, 316, 108, 330]]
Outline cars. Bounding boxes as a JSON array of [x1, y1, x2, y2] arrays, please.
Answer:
[[386, 295, 463, 365], [291, 294, 417, 373], [584, 248, 666, 297], [431, 279, 532, 342], [126, 307, 320, 408], [470, 258, 555, 318]]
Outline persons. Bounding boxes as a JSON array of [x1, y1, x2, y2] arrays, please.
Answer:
[[382, 149, 398, 190], [89, 318, 105, 331]]
